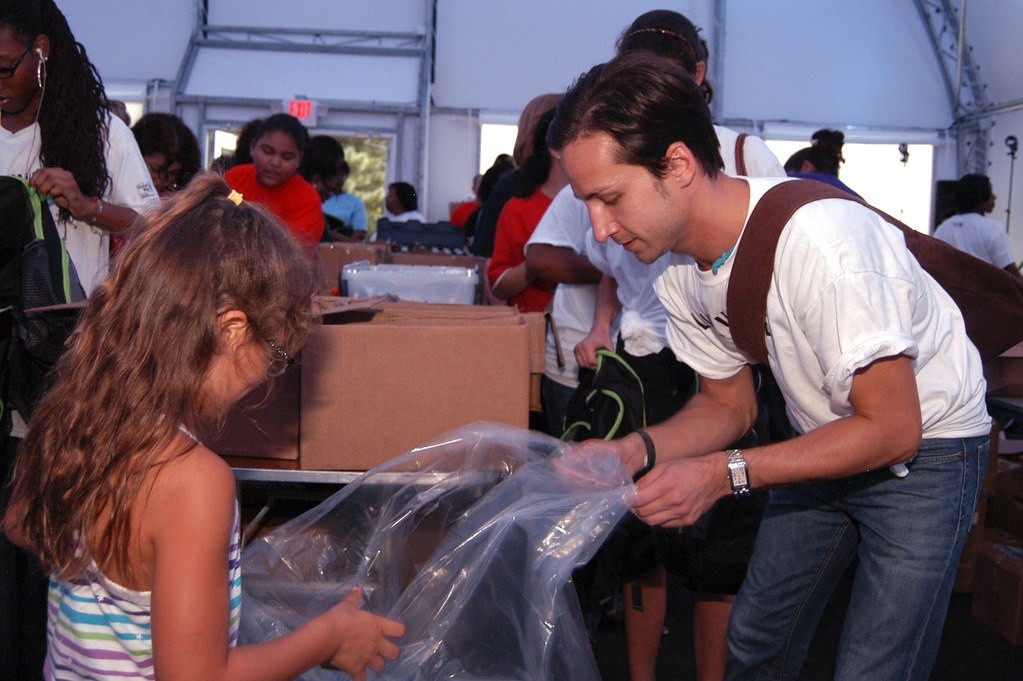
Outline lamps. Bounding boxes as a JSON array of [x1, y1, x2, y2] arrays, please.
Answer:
[[271, 98, 328, 129]]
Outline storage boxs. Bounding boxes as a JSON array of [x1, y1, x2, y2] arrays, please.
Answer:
[[203, 219, 566, 591], [953, 438, 1022, 647], [984, 341, 1022, 398]]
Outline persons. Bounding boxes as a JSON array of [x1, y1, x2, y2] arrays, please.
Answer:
[[932, 173, 1023, 279], [0, 0, 163, 442], [783, 127, 864, 199], [208, 112, 324, 261], [299, 135, 367, 242], [461, 95, 570, 320], [371, 181, 428, 241], [131, 112, 202, 198], [522, 184, 669, 641], [4, 161, 405, 681], [523, 8, 789, 681], [544, 49, 992, 681]]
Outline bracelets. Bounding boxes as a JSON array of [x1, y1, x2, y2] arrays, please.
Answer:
[[632, 428, 655, 472], [85, 196, 102, 224]]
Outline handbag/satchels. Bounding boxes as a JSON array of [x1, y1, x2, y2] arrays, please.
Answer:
[[560, 349, 648, 588], [907, 228, 1023, 360]]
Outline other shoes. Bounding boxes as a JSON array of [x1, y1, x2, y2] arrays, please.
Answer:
[[580, 593, 624, 626]]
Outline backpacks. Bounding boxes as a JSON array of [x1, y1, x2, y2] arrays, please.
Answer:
[[0, 175, 84, 411]]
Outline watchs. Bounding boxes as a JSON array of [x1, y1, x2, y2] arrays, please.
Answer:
[[726, 448, 750, 496]]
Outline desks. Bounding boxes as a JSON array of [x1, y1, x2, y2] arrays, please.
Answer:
[[232, 466, 501, 542], [985, 396, 1022, 495]]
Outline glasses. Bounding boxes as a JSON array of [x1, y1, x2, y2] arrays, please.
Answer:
[[0, 39, 35, 79], [217, 307, 294, 376]]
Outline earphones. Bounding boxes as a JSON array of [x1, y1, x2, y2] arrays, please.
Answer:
[[37, 48, 43, 58]]
